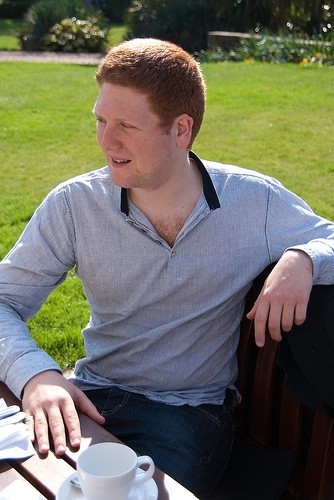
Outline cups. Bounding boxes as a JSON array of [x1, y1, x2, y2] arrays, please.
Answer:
[[76, 442, 155, 500]]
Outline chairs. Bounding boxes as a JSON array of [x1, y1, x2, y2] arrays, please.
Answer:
[[206, 261, 334, 500]]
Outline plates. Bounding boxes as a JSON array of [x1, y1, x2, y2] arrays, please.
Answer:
[[56, 468, 158, 500]]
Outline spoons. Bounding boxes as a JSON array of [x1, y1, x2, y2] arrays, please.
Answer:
[[70, 477, 80, 490]]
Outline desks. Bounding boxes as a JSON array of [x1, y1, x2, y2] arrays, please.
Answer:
[[0, 375, 199, 500]]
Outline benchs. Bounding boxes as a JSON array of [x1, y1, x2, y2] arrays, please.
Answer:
[[209, 32, 334, 53]]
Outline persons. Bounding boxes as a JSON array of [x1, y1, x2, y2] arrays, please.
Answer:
[[0, 38, 334, 500]]
